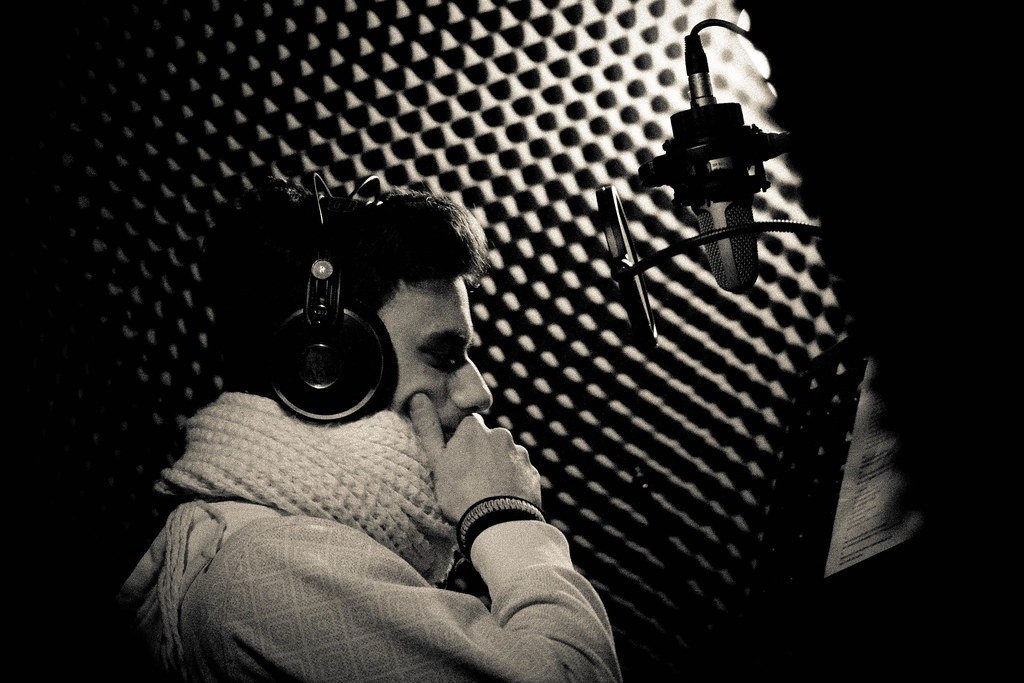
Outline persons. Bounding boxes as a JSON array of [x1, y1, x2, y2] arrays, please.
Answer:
[[118, 175, 621, 683]]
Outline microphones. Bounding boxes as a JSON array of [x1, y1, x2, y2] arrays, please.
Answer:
[[668, 103, 761, 294]]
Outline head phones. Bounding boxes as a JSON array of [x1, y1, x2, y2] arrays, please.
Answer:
[[259, 176, 397, 426]]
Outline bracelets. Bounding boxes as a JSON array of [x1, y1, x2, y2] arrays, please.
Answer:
[[458, 499, 545, 548]]
